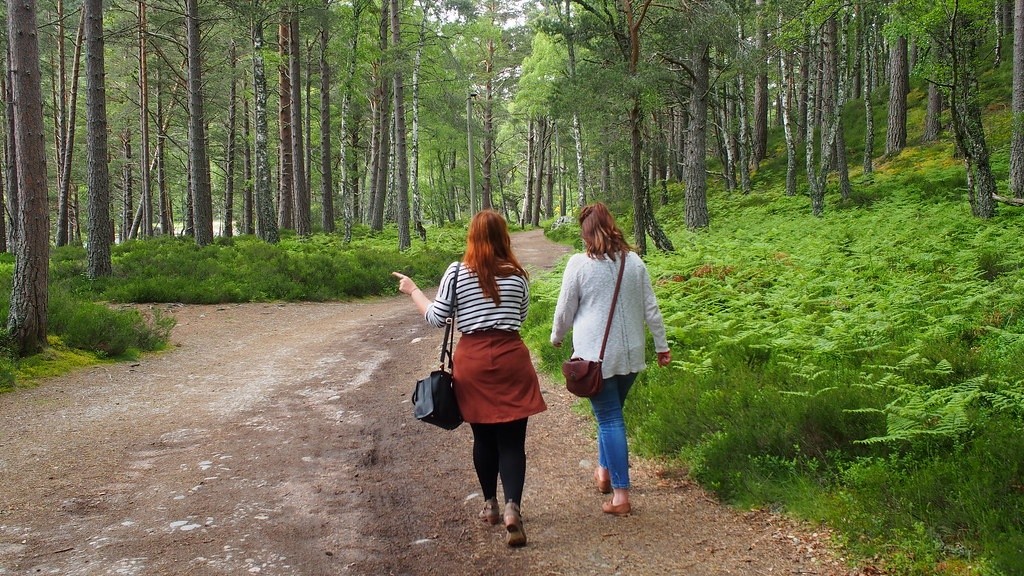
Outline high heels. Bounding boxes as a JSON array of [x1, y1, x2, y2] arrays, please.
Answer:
[[479, 499, 500, 524], [503, 502, 527, 547]]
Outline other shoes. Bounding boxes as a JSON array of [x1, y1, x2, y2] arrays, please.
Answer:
[[602, 501, 631, 516], [594, 467, 609, 492]]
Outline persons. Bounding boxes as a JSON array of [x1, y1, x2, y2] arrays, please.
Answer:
[[549, 202, 671, 514], [392, 209, 547, 548]]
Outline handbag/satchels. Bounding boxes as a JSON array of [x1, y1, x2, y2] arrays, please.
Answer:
[[412, 370, 462, 431], [562, 360, 603, 397]]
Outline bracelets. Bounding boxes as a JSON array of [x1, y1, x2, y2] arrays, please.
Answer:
[[409, 288, 420, 296]]
